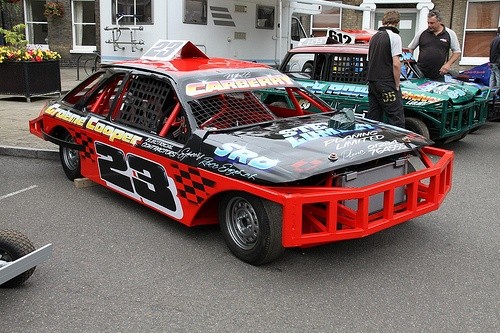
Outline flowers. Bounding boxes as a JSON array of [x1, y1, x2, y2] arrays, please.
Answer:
[[0, 45, 61, 64]]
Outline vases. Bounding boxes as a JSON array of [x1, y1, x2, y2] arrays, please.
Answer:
[[0, 59, 61, 103]]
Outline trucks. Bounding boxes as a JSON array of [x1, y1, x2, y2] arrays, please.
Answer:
[[100, 0, 309, 70]]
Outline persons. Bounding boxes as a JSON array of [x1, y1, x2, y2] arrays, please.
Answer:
[[366, 10, 405, 129], [408, 9, 461, 82], [489, 27, 500, 100]]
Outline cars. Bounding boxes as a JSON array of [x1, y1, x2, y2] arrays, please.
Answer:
[[29, 41, 454, 267], [279, 37, 500, 147]]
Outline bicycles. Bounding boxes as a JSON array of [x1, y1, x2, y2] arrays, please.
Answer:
[[84, 51, 102, 77]]
